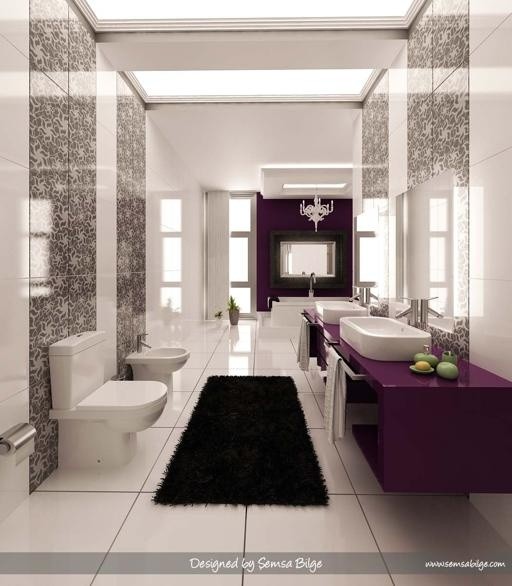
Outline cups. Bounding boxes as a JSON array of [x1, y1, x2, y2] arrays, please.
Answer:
[[442, 350, 456, 365], [302, 272, 305, 277], [285, 272, 289, 276]]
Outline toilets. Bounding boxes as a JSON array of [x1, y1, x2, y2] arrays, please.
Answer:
[[48, 330, 167, 472]]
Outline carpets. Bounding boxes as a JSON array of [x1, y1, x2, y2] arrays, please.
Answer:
[[151, 376, 329, 506]]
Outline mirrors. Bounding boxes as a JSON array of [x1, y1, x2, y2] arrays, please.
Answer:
[[354, 203, 389, 308], [270, 229, 348, 289], [396, 170, 454, 333]]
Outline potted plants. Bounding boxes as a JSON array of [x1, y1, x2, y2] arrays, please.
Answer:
[[215, 311, 224, 327], [228, 296, 239, 325]]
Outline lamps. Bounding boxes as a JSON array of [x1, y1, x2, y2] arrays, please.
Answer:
[[299, 193, 333, 233]]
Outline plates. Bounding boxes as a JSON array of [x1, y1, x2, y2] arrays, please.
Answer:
[[409, 365, 435, 373]]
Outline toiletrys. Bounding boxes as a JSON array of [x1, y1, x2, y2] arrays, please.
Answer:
[[414, 344, 438, 368], [415, 361, 430, 371]]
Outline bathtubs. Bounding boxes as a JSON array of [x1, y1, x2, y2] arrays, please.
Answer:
[[268, 296, 351, 327]]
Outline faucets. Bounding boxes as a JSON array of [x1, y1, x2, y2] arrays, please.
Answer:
[[309, 272, 316, 296], [394, 297, 418, 327], [366, 287, 379, 304], [348, 285, 363, 305], [420, 295, 443, 323], [134, 333, 151, 350]]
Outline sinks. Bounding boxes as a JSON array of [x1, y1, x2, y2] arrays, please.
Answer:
[[126, 346, 190, 375], [340, 316, 432, 362], [315, 301, 368, 325], [429, 316, 454, 334]]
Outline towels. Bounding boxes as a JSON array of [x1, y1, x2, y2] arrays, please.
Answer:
[[269, 297, 279, 308], [324, 346, 347, 443], [296, 317, 310, 371]]
